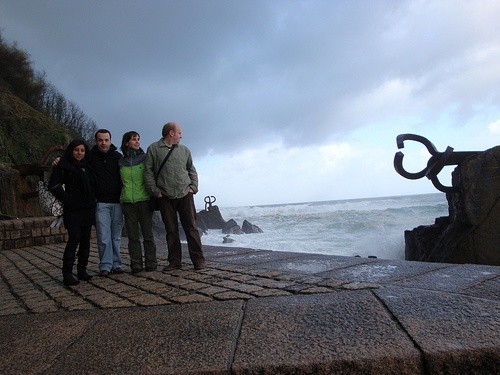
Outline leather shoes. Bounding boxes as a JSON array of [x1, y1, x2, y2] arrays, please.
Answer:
[[99, 270, 111, 276], [193, 261, 206, 270], [113, 266, 125, 274], [170, 260, 182, 269]]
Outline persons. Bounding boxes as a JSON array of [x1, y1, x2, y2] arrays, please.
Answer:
[[47, 140, 97, 286], [144, 122, 205, 273], [119, 131, 159, 273], [52, 129, 124, 276]]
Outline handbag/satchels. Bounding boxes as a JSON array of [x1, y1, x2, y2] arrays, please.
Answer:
[[150, 191, 162, 211], [37, 181, 66, 216]]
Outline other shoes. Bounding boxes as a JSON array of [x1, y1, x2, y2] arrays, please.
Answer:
[[62, 272, 80, 287], [146, 264, 157, 272], [131, 267, 143, 272], [77, 268, 92, 280]]
